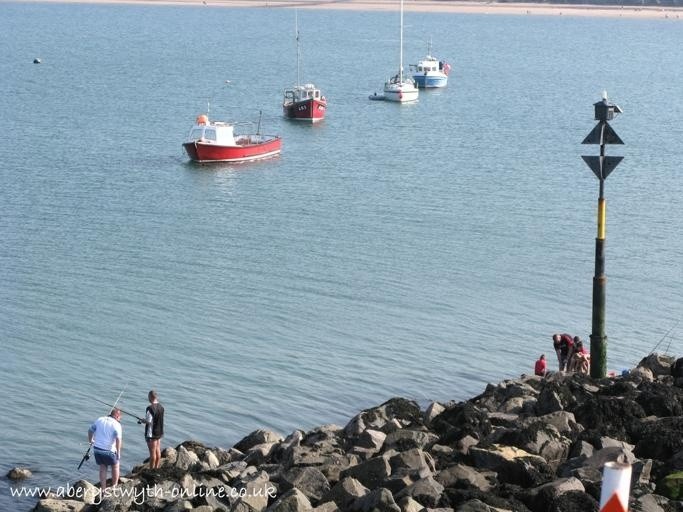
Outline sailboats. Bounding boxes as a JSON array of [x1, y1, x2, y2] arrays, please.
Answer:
[[278, 5, 330, 125], [380, 0, 422, 105]]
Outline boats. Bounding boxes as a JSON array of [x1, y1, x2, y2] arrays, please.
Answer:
[[408, 32, 450, 88], [366, 93, 384, 100], [178, 98, 284, 163]]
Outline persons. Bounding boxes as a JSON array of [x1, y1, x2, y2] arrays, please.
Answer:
[[140, 390, 164, 471], [86, 406, 122, 490], [552, 333, 590, 375], [534, 354, 547, 376]]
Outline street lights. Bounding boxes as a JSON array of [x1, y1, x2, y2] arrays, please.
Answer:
[[588, 86, 624, 383]]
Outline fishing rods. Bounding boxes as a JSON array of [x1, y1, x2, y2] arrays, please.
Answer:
[[77, 383, 146, 470], [647, 321, 677, 356]]
